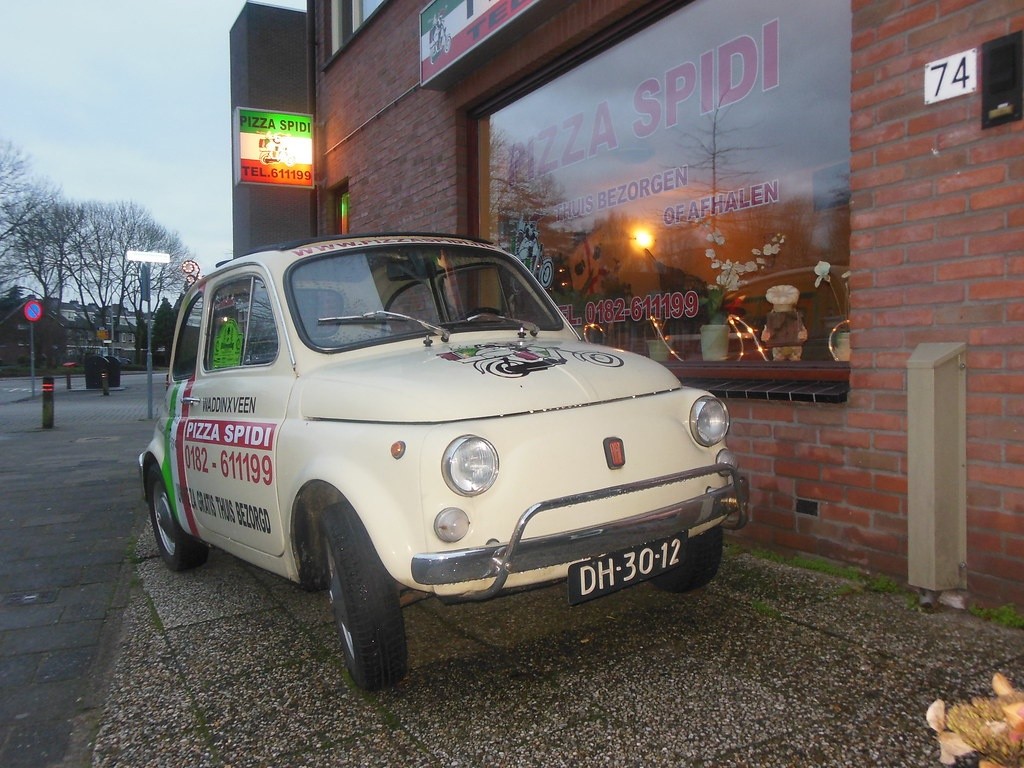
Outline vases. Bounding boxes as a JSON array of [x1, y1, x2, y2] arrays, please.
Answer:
[[697, 323, 732, 361], [827, 319, 852, 362]]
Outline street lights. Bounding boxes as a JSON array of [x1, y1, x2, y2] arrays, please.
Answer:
[[126, 251, 170, 420]]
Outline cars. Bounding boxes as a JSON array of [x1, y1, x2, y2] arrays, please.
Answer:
[[136, 232, 752, 693]]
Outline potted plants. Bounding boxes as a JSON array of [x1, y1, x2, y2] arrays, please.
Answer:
[[636, 287, 677, 364]]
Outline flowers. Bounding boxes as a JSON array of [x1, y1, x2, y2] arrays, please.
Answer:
[[699, 219, 788, 325], [813, 260, 851, 333]]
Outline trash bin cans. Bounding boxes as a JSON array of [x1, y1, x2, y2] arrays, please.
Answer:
[[103, 355, 121, 387], [84, 355, 110, 389]]
